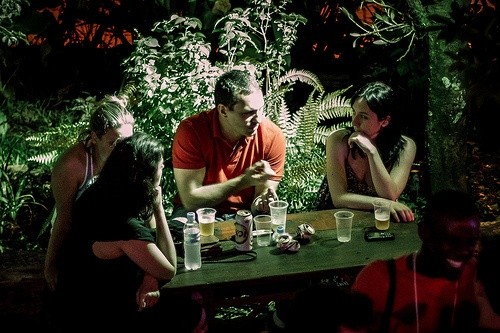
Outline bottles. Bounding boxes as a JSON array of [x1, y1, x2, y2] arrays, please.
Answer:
[[182, 212, 202, 271]]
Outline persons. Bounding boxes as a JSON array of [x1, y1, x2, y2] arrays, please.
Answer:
[[171, 70, 287, 224], [308, 81, 417, 222], [340, 190, 500, 333], [44, 101, 210, 333]]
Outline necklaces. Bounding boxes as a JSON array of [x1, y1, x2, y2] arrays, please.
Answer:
[[413, 254, 458, 333]]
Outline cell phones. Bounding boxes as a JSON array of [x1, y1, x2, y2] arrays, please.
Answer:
[[365, 230, 395, 242]]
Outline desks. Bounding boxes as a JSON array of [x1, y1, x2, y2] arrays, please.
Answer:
[[155, 208, 423, 333]]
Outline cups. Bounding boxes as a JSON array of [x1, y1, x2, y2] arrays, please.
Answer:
[[254, 215, 272, 246], [196, 207, 216, 236], [334, 211, 354, 243], [372, 198, 391, 230], [269, 201, 289, 232]]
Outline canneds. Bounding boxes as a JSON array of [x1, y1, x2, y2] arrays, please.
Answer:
[[234, 210, 253, 251], [276, 235, 300, 252], [297, 223, 315, 244]]
